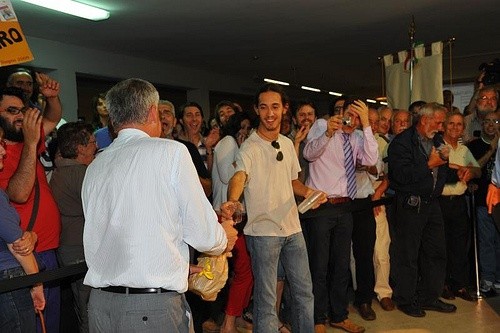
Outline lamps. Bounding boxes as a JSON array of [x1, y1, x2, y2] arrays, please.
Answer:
[[301, 86, 322, 92], [329, 92, 343, 96], [380, 101, 388, 105], [264, 78, 290, 86], [24, 0, 110, 21], [367, 99, 376, 103]]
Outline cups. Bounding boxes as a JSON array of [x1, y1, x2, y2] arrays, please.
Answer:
[[297, 189, 324, 214], [227, 203, 242, 224]]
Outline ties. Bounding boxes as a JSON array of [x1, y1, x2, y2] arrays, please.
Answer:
[[342, 132, 358, 201]]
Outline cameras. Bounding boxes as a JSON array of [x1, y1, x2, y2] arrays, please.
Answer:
[[342, 119, 351, 127]]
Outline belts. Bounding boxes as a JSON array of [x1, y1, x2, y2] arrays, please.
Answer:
[[92, 286, 177, 294], [325, 197, 352, 205]]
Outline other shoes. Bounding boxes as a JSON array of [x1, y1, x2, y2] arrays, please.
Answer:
[[444, 293, 455, 300], [455, 286, 481, 302], [396, 302, 427, 318], [314, 323, 327, 333], [421, 297, 457, 313], [353, 301, 378, 320], [379, 297, 395, 311], [330, 317, 367, 333], [479, 286, 497, 299]]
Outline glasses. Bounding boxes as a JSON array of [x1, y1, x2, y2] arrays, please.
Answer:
[[0, 140, 7, 148], [480, 96, 497, 101], [2, 106, 28, 115], [335, 107, 345, 112], [88, 141, 97, 145], [272, 140, 284, 162]]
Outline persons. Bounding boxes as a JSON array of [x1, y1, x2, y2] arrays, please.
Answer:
[[219, 85, 328, 333], [1, 64, 500, 333], [304, 90, 380, 333], [78, 78, 239, 332]]
